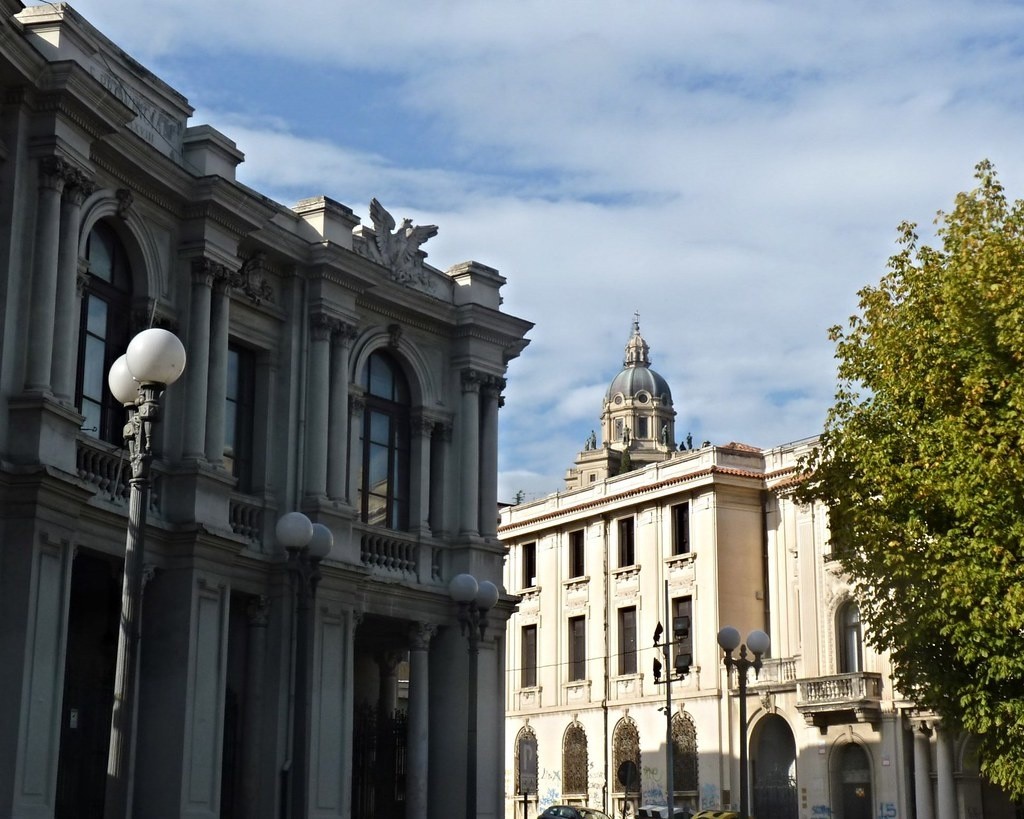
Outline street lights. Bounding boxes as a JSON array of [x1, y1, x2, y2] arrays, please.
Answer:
[[276, 512, 333, 819], [99, 326, 189, 819], [716, 626, 771, 819], [447, 574, 499, 819]]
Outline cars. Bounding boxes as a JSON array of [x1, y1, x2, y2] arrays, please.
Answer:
[[537, 805, 611, 819]]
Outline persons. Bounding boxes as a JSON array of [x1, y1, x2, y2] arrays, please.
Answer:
[[661, 425, 669, 446], [584, 439, 589, 451], [591, 430, 597, 449], [687, 432, 693, 449], [623, 425, 630, 444], [680, 441, 686, 451]]
